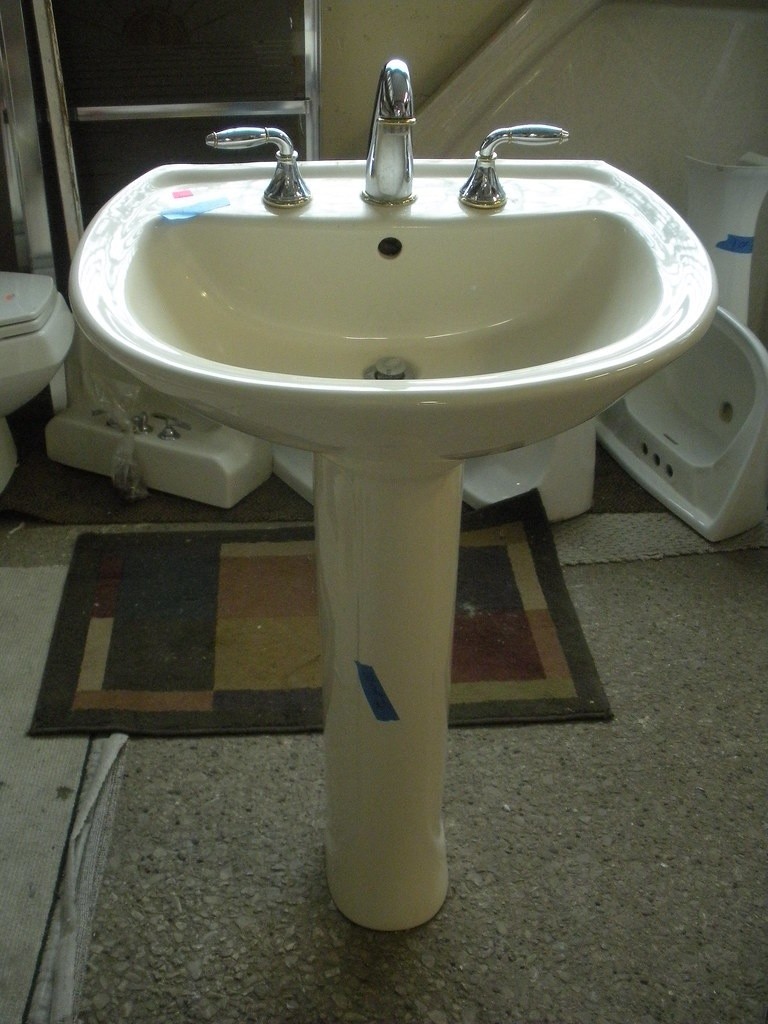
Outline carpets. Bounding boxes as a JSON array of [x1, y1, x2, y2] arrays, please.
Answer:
[[27, 488, 612, 735], [0, 406, 669, 525]]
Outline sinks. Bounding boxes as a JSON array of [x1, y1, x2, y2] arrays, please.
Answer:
[[596, 304, 768, 543], [67, 157, 718, 461]]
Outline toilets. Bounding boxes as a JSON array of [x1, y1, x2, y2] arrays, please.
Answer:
[[0, 269, 76, 495]]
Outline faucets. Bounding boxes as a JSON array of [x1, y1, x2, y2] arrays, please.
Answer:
[[120, 410, 153, 433], [359, 57, 417, 205]]
